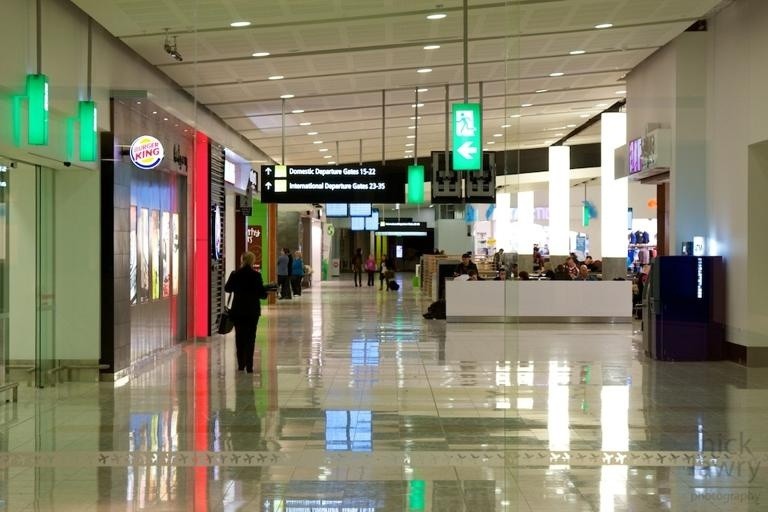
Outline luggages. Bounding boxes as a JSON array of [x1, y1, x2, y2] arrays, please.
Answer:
[[389, 280, 400, 291]]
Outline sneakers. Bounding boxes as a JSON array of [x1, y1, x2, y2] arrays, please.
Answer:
[[277, 292, 302, 300], [355, 282, 389, 291], [238, 363, 254, 374]]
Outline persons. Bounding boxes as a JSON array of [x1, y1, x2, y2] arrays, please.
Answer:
[[453, 243, 599, 281], [364, 253, 378, 289], [228, 371, 263, 485], [276, 248, 304, 300], [351, 248, 365, 288], [378, 254, 398, 291], [224, 251, 268, 373]]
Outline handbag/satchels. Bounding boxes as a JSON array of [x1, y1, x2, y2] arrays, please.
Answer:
[[384, 270, 395, 279], [218, 305, 233, 335]]
